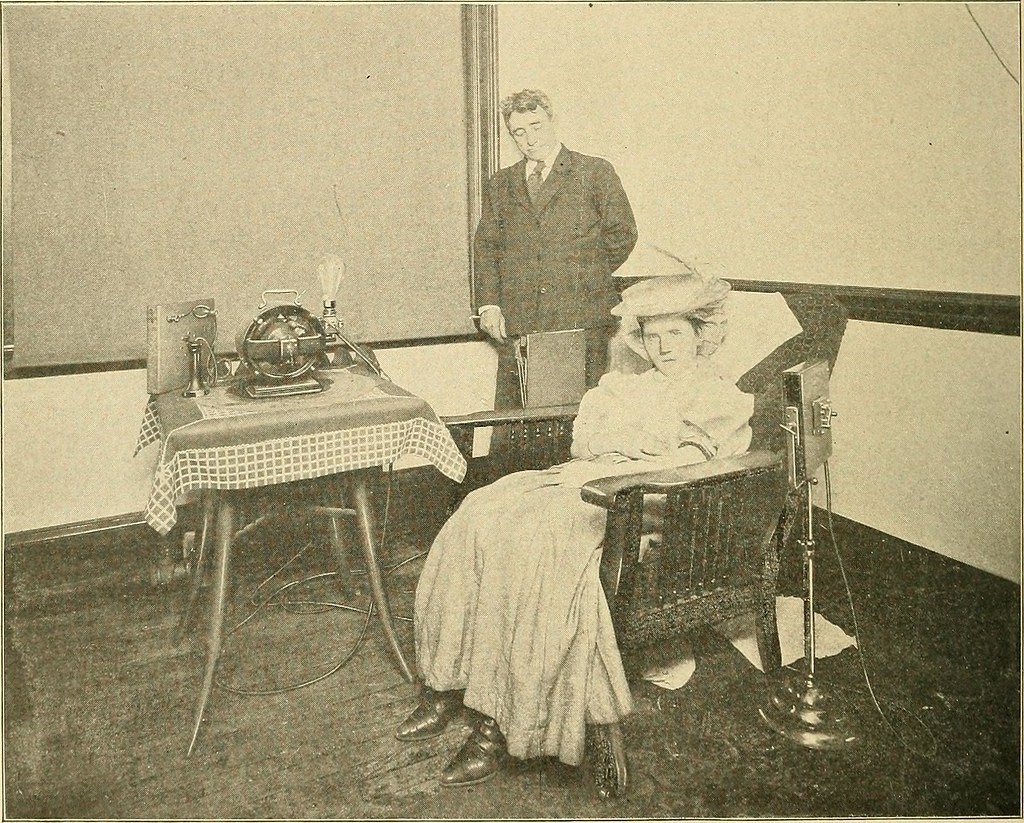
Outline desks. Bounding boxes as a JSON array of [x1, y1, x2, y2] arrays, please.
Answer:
[[137, 352, 467, 760]]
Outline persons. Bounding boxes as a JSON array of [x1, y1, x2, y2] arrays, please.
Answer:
[[391, 275, 755, 788], [471, 88, 638, 464]]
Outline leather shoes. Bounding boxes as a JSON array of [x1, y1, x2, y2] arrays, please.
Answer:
[[436, 710, 508, 791], [394, 681, 467, 745]]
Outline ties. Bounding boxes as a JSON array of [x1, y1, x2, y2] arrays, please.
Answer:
[[524, 161, 548, 210]]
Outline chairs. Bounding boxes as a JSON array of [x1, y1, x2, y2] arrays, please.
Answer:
[[443, 280, 849, 678]]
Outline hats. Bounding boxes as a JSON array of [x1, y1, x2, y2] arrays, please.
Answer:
[[611, 271, 732, 317]]
